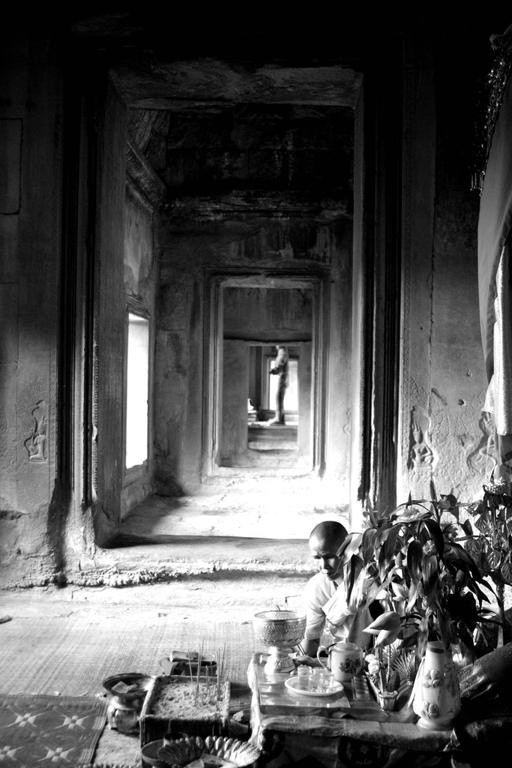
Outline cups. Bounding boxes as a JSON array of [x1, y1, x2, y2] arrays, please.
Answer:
[[297, 666, 337, 693]]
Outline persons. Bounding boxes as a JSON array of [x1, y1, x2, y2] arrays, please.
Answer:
[[284, 520, 390, 659], [269, 344, 290, 426]]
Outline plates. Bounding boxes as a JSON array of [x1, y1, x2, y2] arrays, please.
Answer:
[[284, 677, 344, 696]]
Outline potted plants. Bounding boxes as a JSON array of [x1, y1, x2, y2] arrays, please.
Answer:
[[336, 479, 503, 731]]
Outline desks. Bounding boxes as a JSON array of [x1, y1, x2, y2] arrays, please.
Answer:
[[246, 652, 512, 768]]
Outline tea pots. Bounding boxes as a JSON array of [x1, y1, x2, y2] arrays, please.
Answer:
[[317, 638, 367, 684]]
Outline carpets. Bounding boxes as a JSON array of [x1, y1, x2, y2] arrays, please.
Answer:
[[0, 694, 110, 768]]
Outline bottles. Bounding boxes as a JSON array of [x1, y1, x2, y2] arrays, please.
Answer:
[[411, 647, 460, 731]]
